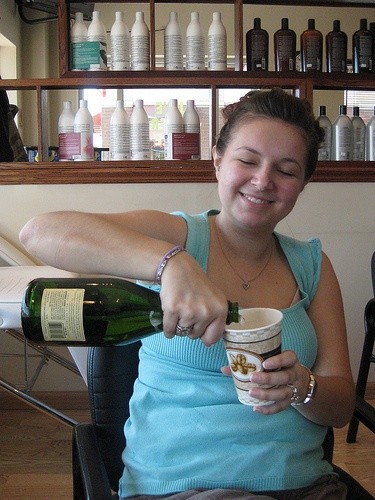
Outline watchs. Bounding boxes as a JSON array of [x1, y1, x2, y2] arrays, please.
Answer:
[[291, 364, 318, 407]]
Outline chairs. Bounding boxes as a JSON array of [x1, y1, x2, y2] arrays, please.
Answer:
[[0, 238, 375, 500]]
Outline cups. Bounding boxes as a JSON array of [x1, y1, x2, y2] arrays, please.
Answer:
[[222, 307, 285, 407]]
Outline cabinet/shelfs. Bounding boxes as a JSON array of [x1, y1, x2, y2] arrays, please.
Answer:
[[0, 0, 375, 184]]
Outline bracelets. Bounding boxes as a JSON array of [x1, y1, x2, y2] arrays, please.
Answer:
[[156, 246, 186, 282]]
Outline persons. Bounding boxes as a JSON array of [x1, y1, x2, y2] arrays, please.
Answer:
[[18, 87, 348, 500]]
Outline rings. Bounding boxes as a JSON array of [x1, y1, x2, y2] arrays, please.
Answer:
[[287, 384, 300, 403], [176, 324, 193, 335]]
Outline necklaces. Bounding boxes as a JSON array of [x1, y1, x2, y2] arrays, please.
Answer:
[[215, 223, 273, 291]]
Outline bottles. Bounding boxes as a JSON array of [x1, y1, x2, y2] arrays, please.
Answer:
[[73, 99, 94, 161], [181, 100, 202, 160], [208, 12, 227, 71], [19, 277, 239, 347], [246, 18, 269, 71], [185, 12, 205, 71], [351, 18, 375, 73], [273, 18, 296, 71], [163, 12, 182, 70], [326, 20, 347, 72], [58, 101, 74, 161], [300, 19, 323, 72], [163, 99, 183, 160], [70, 12, 87, 71], [109, 100, 129, 161], [110, 11, 129, 71], [131, 12, 150, 71], [316, 105, 374, 161], [87, 11, 107, 71], [129, 99, 152, 160]]
